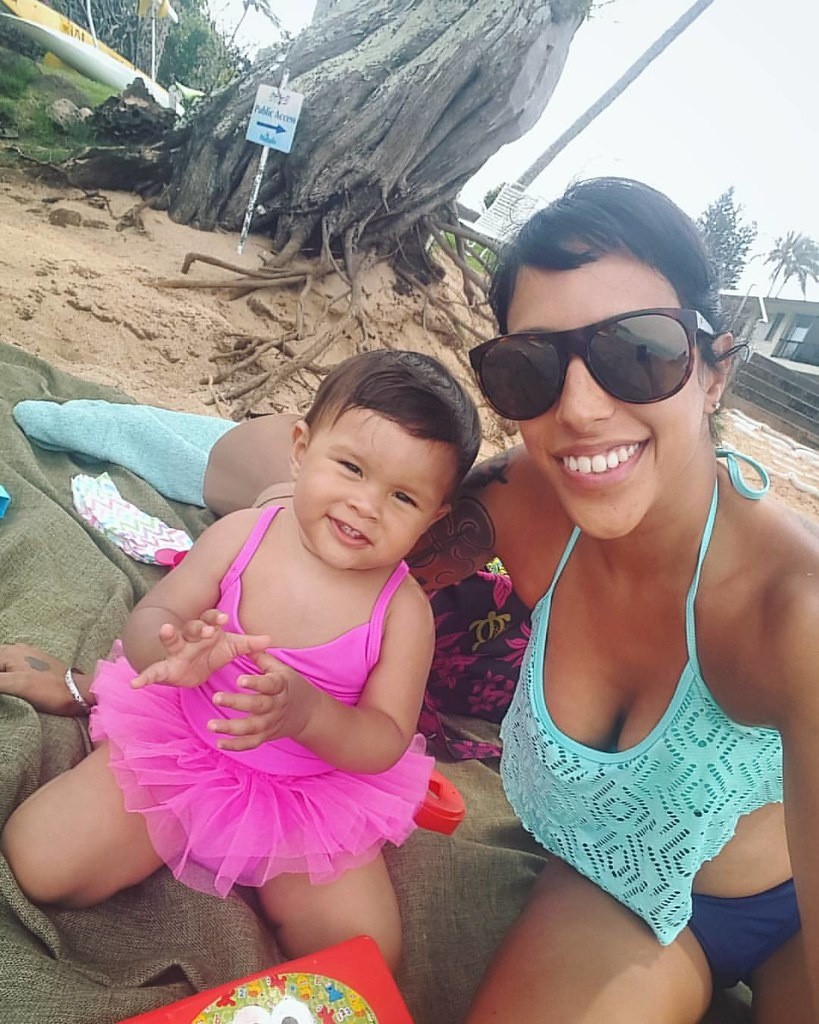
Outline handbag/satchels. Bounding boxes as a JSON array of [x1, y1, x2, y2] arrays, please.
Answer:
[[424, 567, 532, 759]]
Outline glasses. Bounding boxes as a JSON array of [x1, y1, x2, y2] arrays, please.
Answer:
[[467, 307, 713, 419]]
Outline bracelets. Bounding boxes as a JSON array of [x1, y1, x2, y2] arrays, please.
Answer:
[[65, 667, 92, 714]]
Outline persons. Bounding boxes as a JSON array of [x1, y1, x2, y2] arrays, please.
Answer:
[[0, 177, 819, 1024], [0, 349, 482, 974]]
